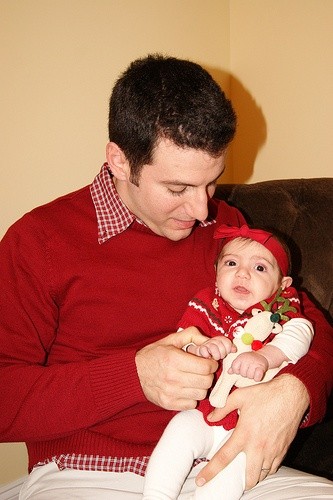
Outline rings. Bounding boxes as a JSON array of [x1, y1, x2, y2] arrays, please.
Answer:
[[262, 468, 270, 472]]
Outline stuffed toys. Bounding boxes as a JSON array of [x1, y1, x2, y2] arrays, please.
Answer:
[[209, 289, 297, 408]]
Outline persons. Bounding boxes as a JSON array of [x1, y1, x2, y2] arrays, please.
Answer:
[[0, 53, 333, 500], [142, 225, 314, 500]]
